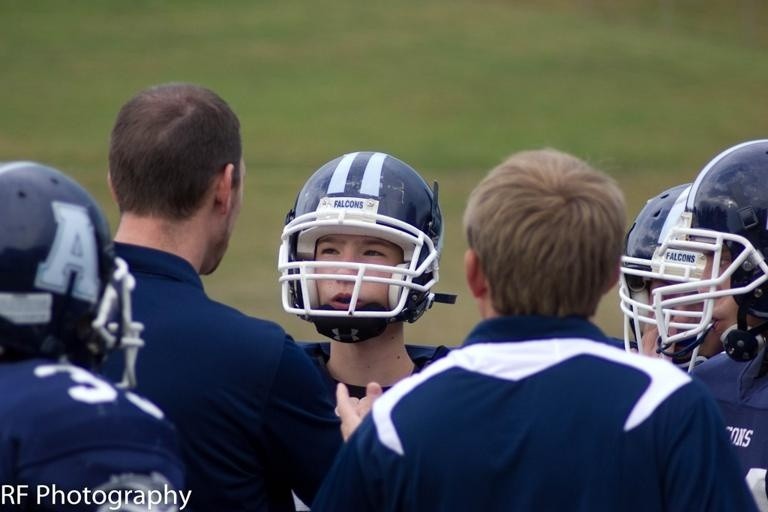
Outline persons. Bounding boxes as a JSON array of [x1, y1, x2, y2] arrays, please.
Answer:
[[277, 147, 450, 509], [652, 138, 768, 511], [107, 83, 345, 511], [1, 158, 215, 510], [307, 145, 760, 510], [616, 183, 724, 371]]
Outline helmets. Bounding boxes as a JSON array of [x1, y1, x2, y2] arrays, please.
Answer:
[[0, 160, 146, 391], [617, 138, 764, 372], [278, 151, 444, 343]]
